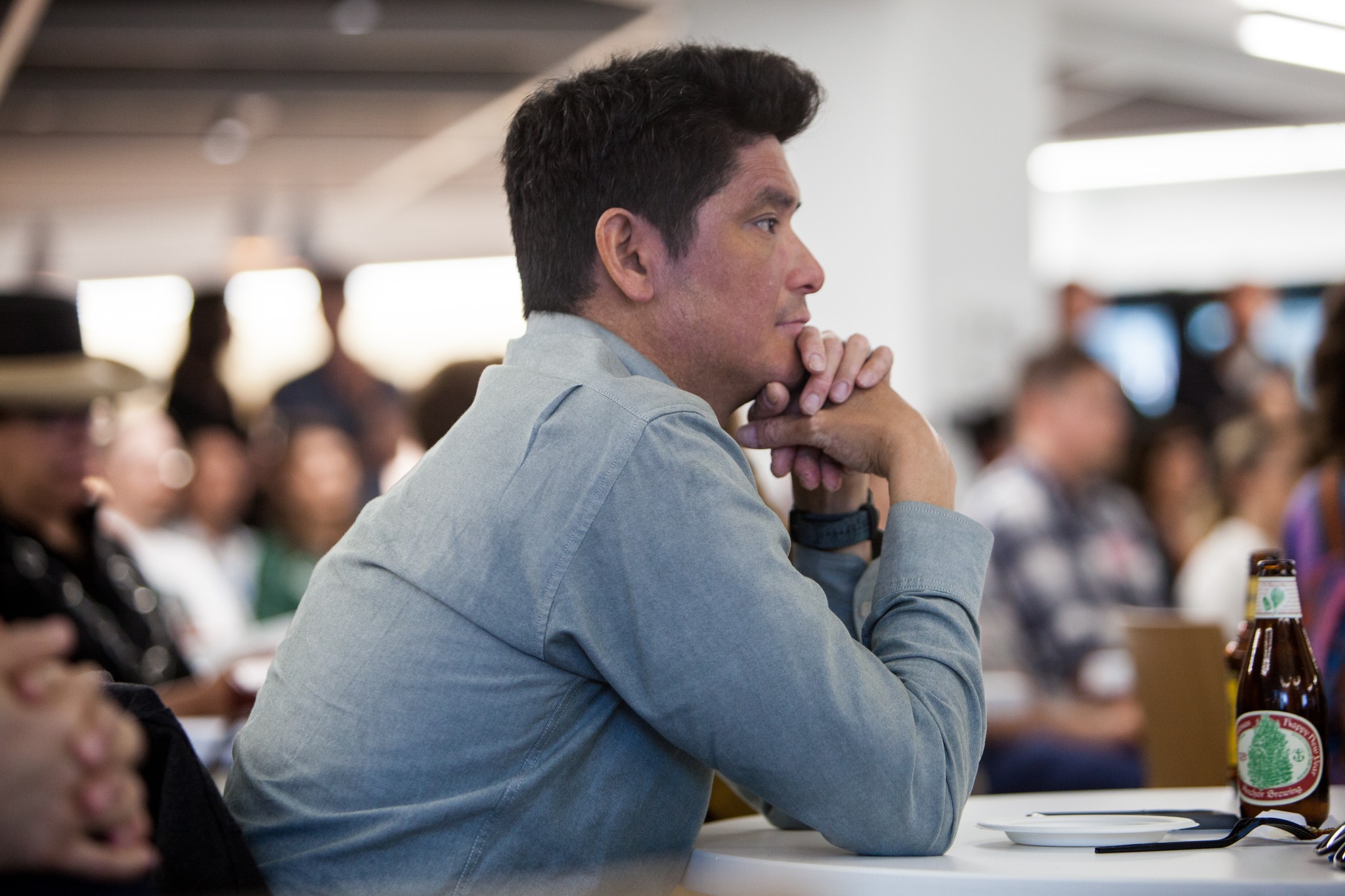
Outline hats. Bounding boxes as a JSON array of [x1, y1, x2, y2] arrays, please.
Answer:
[[0, 291, 146, 409]]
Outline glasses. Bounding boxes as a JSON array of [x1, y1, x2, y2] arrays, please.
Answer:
[[1314, 822, 1345, 867]]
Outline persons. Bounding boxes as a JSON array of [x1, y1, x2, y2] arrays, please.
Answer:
[[222, 42, 994, 896], [0, 272, 1345, 896]]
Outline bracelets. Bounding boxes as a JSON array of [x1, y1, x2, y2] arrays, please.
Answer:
[[788, 487, 881, 550]]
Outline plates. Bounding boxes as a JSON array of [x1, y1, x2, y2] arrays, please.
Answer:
[[976, 815, 1200, 847]]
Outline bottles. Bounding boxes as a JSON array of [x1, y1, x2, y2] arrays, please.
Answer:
[[1236, 559, 1329, 829], [1223, 550, 1286, 796]]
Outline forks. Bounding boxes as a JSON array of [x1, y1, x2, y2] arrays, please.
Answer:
[[1095, 818, 1317, 854]]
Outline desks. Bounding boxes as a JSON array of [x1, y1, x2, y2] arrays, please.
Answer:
[[681, 786, 1345, 896]]
